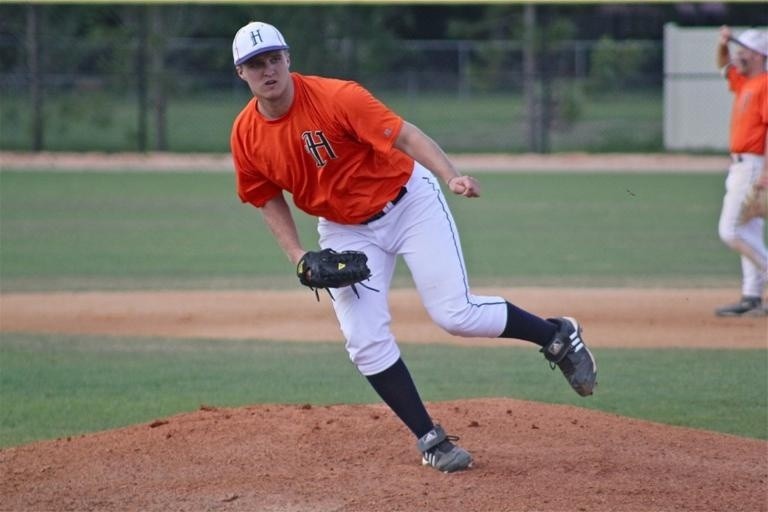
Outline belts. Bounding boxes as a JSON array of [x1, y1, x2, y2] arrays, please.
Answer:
[[358, 185, 407, 225], [738, 153, 743, 163]]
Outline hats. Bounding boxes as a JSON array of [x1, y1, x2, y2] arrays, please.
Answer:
[[232, 20, 289, 66], [728, 28, 768, 57]]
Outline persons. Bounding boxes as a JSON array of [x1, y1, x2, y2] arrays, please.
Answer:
[[713, 25, 768, 316], [231, 21, 600, 471]]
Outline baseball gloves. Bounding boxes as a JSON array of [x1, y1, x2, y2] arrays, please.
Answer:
[[734, 184, 766, 228], [296, 248, 372, 288]]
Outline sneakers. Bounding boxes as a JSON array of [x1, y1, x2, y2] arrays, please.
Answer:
[[715, 295, 762, 317], [539, 316, 598, 397], [417, 423, 473, 473]]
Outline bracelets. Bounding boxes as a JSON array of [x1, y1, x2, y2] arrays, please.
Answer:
[[444, 173, 461, 189]]
